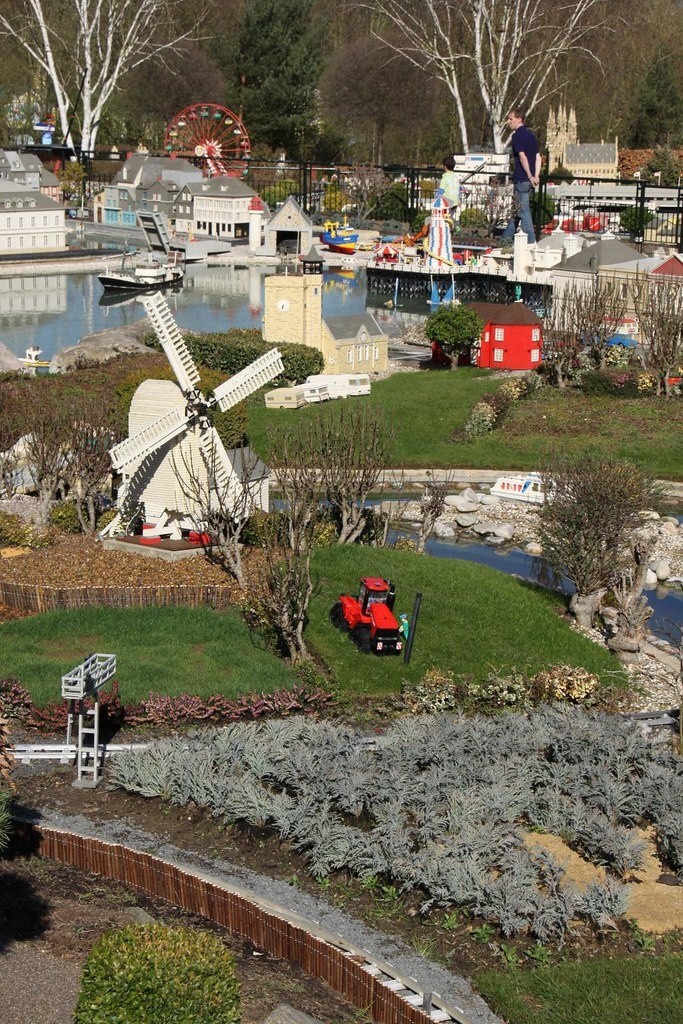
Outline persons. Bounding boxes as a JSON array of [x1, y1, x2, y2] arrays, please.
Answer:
[[439, 156, 462, 236], [399, 614, 409, 639], [499, 108, 542, 247]]
